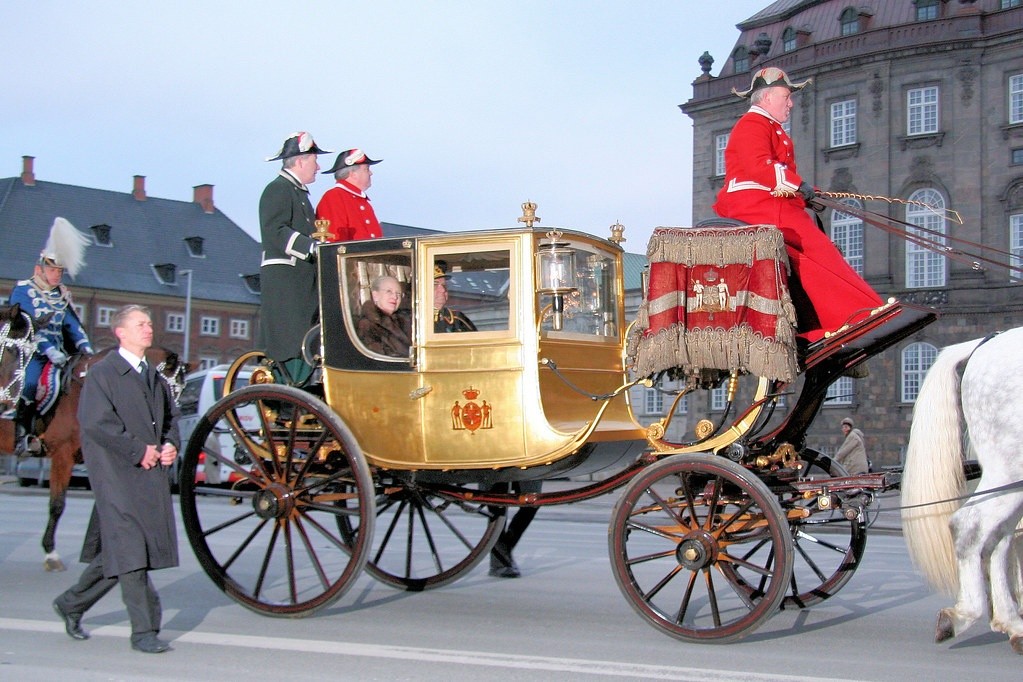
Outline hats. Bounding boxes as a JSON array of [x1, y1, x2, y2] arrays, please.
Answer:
[[841, 418, 854, 428], [321, 149, 383, 174], [731, 67, 812, 99], [434, 260, 452, 280], [39, 254, 65, 268], [264, 131, 335, 162]]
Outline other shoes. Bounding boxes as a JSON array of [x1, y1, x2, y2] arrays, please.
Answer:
[[275, 408, 318, 427], [488, 562, 521, 576]]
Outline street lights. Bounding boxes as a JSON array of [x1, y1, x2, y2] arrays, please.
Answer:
[[179, 269, 193, 364]]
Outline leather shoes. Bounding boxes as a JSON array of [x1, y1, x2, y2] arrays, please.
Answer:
[[53, 597, 88, 640], [131, 635, 169, 653]]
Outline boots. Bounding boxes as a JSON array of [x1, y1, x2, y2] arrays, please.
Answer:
[[13, 395, 37, 455]]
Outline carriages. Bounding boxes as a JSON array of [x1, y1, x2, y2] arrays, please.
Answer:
[[178, 199, 1023, 659]]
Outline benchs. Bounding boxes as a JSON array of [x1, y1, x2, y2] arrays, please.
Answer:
[[693, 218, 748, 229]]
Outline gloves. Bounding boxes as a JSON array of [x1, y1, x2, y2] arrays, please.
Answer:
[[812, 196, 827, 213], [85, 347, 94, 355], [45, 348, 66, 367], [797, 182, 820, 200]]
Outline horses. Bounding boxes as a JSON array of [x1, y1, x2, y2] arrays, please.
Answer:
[[0, 303, 204, 572], [899, 325, 1023, 657]]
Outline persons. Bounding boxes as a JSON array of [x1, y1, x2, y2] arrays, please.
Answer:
[[9, 216, 95, 457], [833, 417, 869, 475], [52, 305, 181, 653], [485, 479, 540, 578], [259, 131, 478, 421], [711, 66, 885, 343]]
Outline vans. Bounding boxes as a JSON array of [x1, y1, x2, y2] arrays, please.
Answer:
[[174, 364, 282, 488]]
